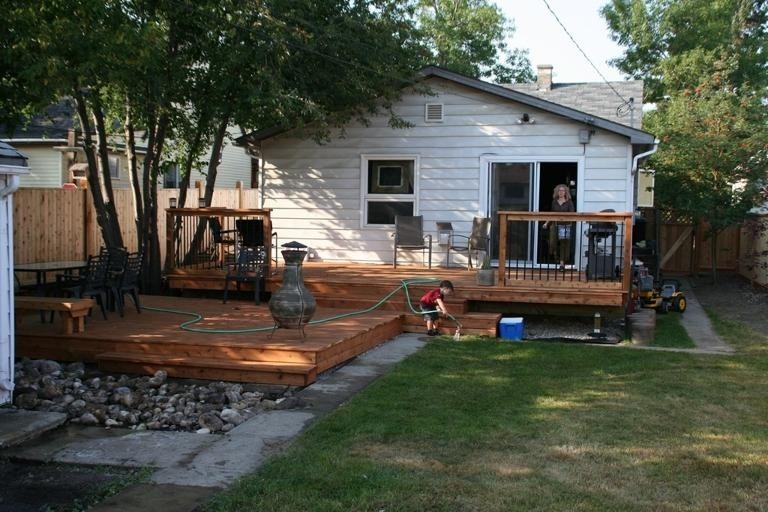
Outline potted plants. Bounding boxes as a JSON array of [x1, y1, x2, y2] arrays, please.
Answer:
[[475, 253, 495, 286]]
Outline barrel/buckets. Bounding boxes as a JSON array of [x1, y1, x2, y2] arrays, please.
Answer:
[[627, 307, 655, 344]]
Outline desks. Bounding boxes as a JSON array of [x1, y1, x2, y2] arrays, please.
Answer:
[[14, 259, 101, 323]]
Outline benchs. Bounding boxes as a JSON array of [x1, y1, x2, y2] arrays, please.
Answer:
[[14, 295, 96, 335]]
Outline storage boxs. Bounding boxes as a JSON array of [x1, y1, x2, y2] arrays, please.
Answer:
[[498, 315, 524, 340]]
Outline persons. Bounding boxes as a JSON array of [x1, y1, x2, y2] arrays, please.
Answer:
[[542, 184, 576, 273], [419, 279, 454, 336]]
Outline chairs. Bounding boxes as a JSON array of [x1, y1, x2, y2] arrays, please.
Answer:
[[392, 214, 435, 270], [208, 217, 271, 304], [447, 217, 493, 271], [54, 246, 143, 319]]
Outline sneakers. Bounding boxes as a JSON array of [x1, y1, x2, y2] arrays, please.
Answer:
[[559, 266, 564, 272], [427, 330, 441, 336]]
